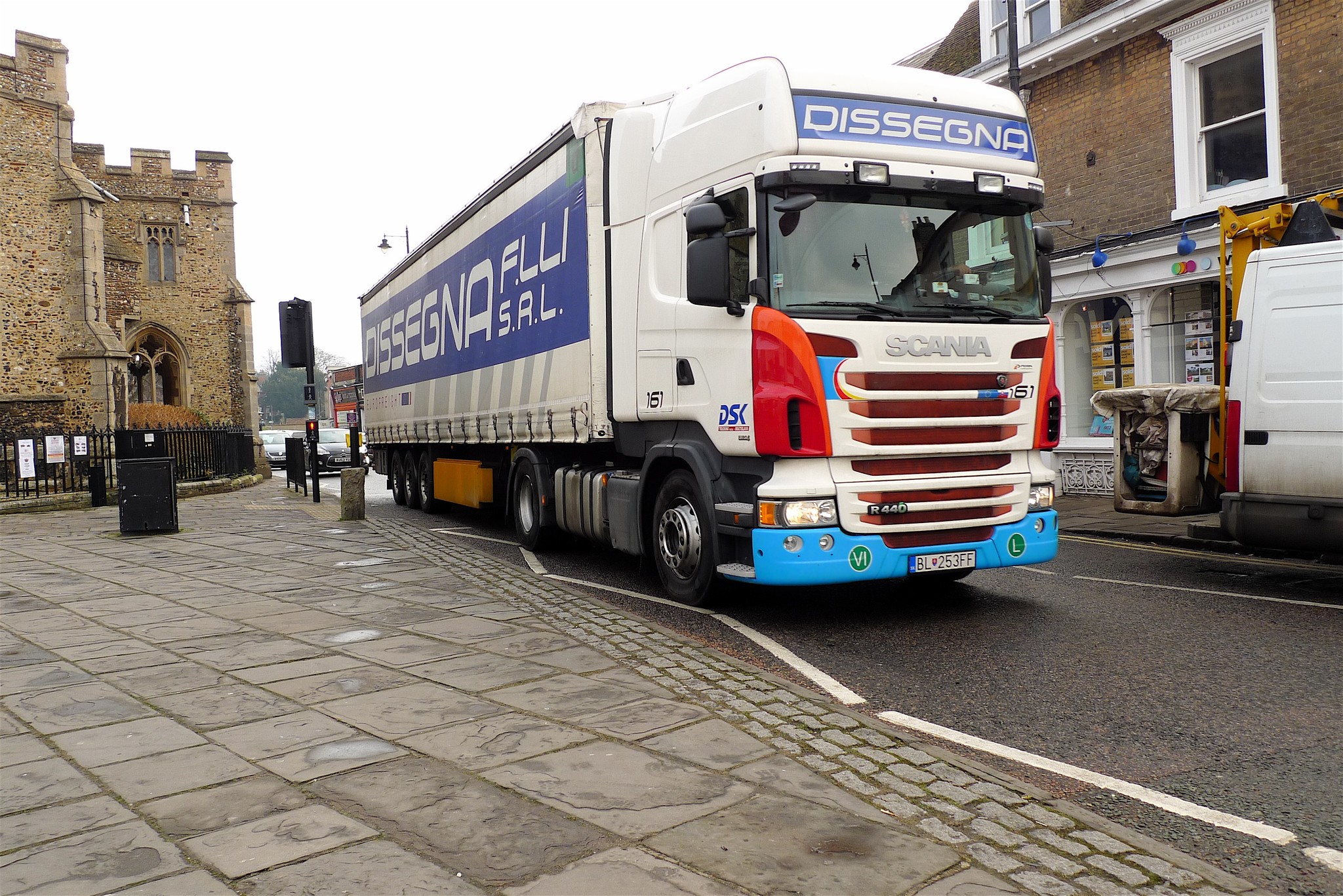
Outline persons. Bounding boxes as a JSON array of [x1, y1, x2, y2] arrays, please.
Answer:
[[905, 237, 971, 288]]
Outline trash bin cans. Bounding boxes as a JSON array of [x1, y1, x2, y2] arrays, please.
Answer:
[[89, 465, 107, 507], [115, 457, 182, 536]]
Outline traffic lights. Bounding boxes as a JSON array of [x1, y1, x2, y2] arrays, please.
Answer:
[[304, 383, 317, 405], [306, 419, 319, 442]]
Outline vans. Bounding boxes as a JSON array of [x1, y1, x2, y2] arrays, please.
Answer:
[[1217, 238, 1342, 564]]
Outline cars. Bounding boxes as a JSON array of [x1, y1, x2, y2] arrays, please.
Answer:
[[258, 428, 374, 475]]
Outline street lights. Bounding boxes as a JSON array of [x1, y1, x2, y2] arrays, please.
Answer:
[[377, 224, 409, 256]]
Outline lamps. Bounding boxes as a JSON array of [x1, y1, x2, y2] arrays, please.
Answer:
[[1177, 213, 1219, 256], [1092, 232, 1133, 267]]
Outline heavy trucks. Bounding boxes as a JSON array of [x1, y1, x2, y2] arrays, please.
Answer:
[[356, 54, 1062, 606]]
